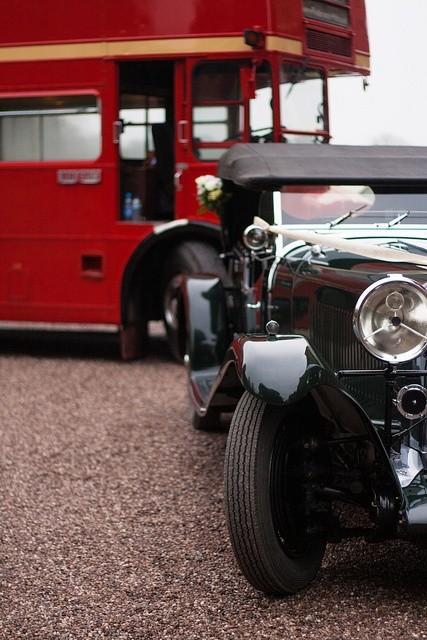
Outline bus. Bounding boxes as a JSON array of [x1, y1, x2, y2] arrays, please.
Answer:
[[1, 0, 371, 361]]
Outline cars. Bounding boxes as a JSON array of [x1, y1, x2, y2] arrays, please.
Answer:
[[176, 144, 427, 594]]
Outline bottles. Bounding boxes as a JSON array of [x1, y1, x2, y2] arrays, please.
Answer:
[[123, 192, 134, 221], [131, 196, 142, 221]]
